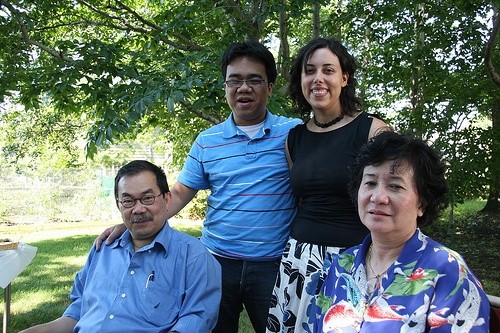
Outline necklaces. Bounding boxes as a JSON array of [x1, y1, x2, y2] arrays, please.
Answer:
[[367, 241, 411, 280], [312, 110, 345, 129]]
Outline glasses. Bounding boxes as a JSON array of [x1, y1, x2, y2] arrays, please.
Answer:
[[223, 76, 267, 88], [116, 192, 164, 208]]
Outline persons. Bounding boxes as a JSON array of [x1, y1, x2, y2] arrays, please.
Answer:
[[15, 158, 222, 333], [292, 124, 492, 333], [264, 38, 393, 333], [93, 41, 305, 333]]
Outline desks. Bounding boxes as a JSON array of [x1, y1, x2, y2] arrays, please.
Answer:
[[0, 243, 38, 333]]
